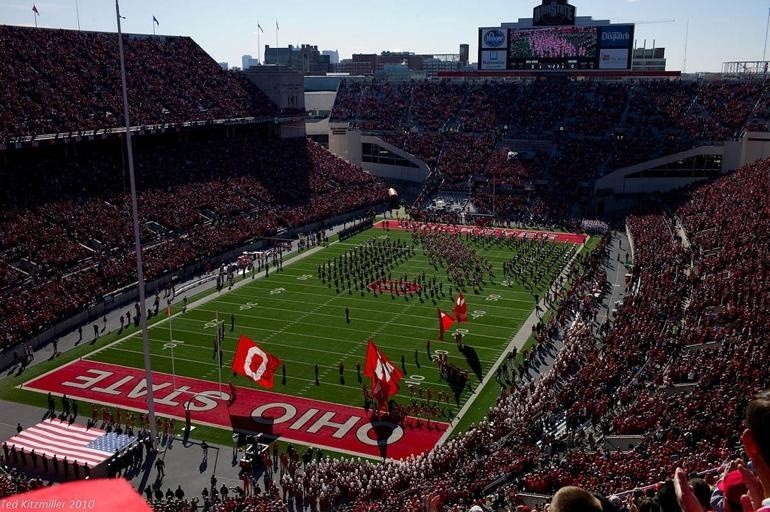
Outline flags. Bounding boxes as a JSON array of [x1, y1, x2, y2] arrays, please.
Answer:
[[275, 19, 280, 31], [257, 20, 263, 31], [152, 15, 159, 26], [31, 2, 40, 17]]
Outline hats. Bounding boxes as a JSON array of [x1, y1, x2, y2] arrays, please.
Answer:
[[717, 468, 751, 505]]
